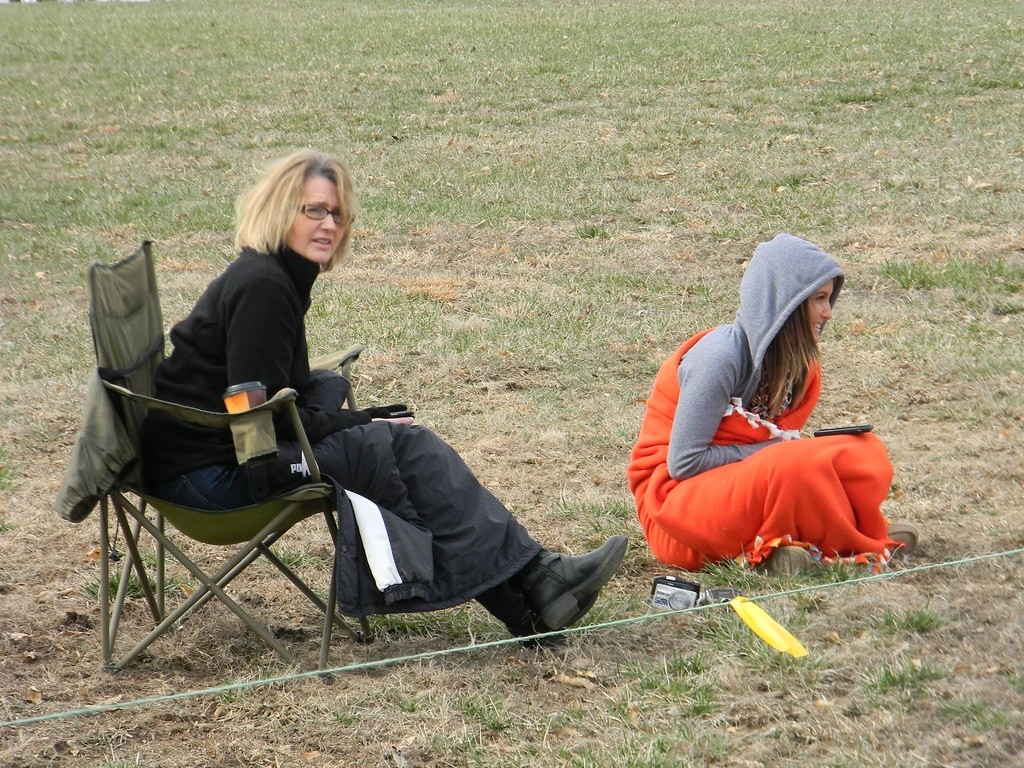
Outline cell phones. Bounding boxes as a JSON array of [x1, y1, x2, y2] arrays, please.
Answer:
[[814, 425, 872, 436], [389, 411, 414, 416]]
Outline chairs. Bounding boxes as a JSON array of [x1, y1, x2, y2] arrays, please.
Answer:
[[54, 241, 375, 679]]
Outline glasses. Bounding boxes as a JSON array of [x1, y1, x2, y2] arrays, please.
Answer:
[[289, 204, 341, 224]]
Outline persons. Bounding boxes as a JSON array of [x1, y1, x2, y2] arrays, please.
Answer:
[[139, 150, 629, 652], [626, 231, 919, 577]]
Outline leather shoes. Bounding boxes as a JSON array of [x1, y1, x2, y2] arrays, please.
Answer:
[[523, 535, 630, 631], [497, 590, 599, 647]]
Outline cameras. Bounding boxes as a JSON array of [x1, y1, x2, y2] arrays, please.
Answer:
[[650, 575, 700, 612]]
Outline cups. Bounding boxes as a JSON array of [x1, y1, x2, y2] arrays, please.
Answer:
[[223, 381, 268, 418]]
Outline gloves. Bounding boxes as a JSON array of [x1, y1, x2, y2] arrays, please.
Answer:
[[361, 405, 416, 418]]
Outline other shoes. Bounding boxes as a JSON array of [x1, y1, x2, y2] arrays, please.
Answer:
[[883, 524, 919, 552], [773, 546, 812, 575]]
